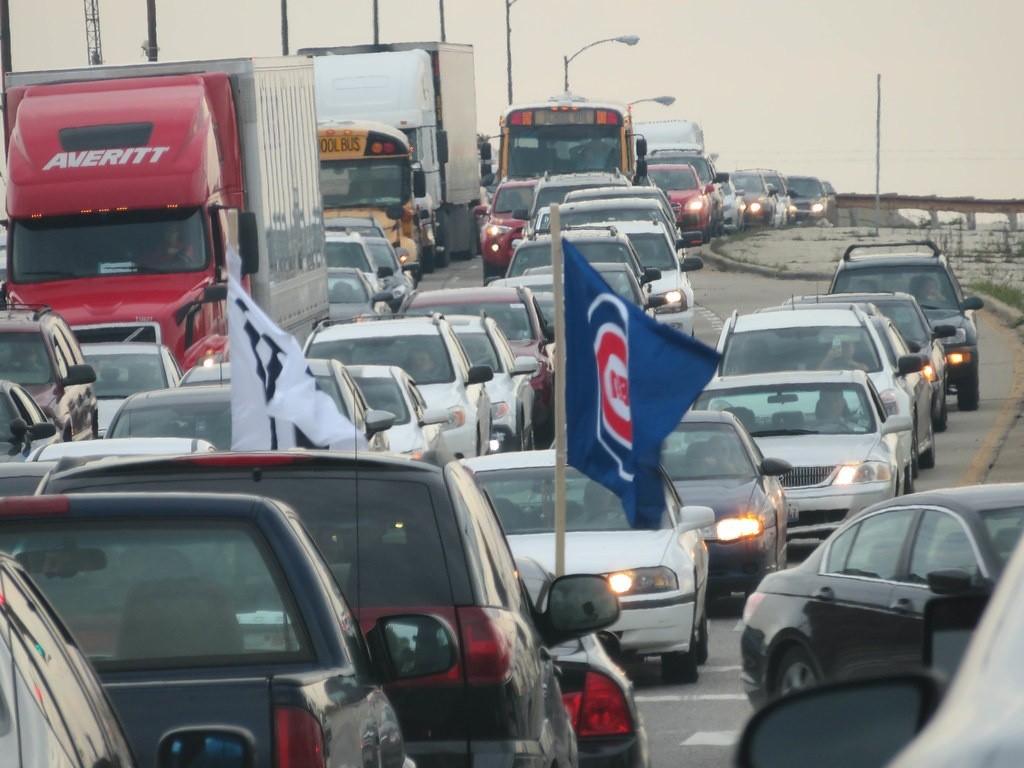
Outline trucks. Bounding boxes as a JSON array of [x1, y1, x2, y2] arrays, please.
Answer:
[[1, 54, 334, 376]]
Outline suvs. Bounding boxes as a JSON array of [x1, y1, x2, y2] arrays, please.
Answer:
[[511, 168, 632, 231], [0, 303, 102, 447], [828, 241, 984, 411], [486, 223, 661, 294]]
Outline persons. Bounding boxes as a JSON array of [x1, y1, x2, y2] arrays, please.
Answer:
[[408, 347, 435, 375], [692, 435, 739, 477], [144, 227, 195, 264], [805, 389, 864, 432], [566, 477, 625, 528], [917, 277, 947, 305], [816, 337, 869, 371], [569, 144, 613, 172], [0, 347, 44, 370]]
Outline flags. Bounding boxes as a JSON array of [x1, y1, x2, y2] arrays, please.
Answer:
[[562, 236, 725, 530]]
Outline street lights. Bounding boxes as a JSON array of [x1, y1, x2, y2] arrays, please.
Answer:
[[563, 35, 639, 94]]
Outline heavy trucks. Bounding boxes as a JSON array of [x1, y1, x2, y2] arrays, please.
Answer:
[[297, 40, 483, 266]]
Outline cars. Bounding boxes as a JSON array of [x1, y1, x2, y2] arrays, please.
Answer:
[[508, 553, 652, 768], [2, 124, 961, 687], [37, 447, 622, 768], [738, 482, 1023, 713], [0, 547, 256, 768], [728, 528, 1024, 767], [0, 492, 460, 768]]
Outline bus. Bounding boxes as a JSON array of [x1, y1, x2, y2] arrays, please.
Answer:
[[315, 118, 427, 265], [480, 102, 650, 184]]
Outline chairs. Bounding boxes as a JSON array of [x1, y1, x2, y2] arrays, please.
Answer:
[[123, 362, 153, 393], [934, 529, 976, 571], [94, 367, 118, 391], [866, 540, 903, 577], [119, 576, 244, 662], [995, 527, 1021, 559], [725, 407, 758, 433], [774, 410, 809, 428]]
